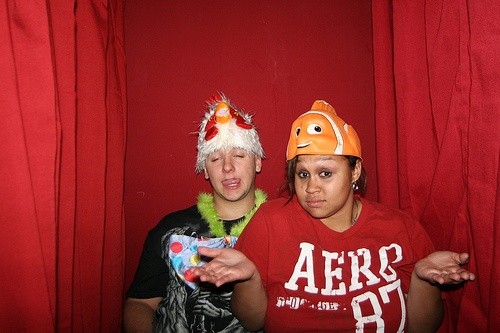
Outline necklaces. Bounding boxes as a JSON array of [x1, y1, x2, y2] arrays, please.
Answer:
[[353, 199, 358, 225], [197, 188, 267, 238]]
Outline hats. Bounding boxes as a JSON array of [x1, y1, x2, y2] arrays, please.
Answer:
[[286, 100, 365, 162], [194, 95, 265, 173]]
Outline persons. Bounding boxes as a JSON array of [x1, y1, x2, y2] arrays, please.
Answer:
[[121, 91, 269, 333], [190, 100, 475, 333]]
